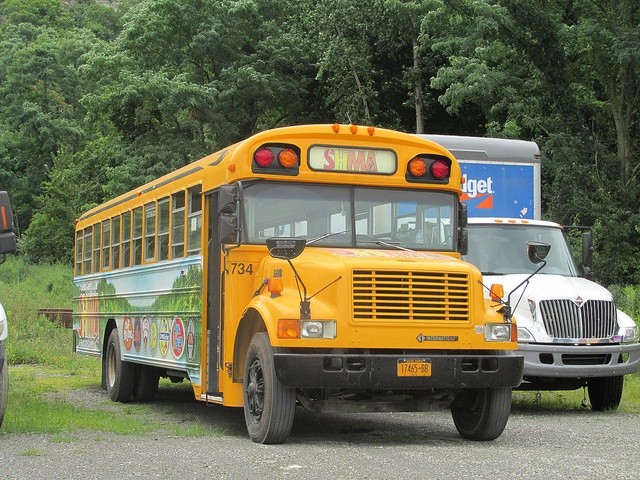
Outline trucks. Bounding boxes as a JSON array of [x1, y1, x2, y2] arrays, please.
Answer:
[[256, 133, 640, 410]]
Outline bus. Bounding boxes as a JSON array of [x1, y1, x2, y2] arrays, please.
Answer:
[[72, 123, 552, 444]]
